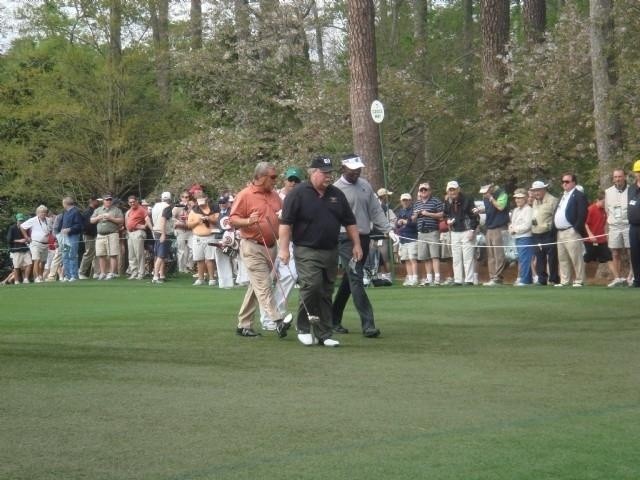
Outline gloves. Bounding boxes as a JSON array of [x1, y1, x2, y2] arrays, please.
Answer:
[[389, 229, 401, 247]]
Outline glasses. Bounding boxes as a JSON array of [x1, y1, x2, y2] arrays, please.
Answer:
[[288, 175, 302, 184], [561, 179, 574, 184], [420, 188, 428, 192], [261, 173, 279, 180]]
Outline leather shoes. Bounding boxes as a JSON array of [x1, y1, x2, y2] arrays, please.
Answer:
[[274, 320, 293, 338], [362, 327, 381, 338], [332, 324, 349, 335], [235, 326, 261, 337]]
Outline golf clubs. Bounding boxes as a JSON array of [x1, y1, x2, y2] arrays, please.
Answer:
[[266, 216, 320, 322], [256, 222, 293, 324]]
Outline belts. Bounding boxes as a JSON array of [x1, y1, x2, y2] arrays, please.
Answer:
[[33, 240, 50, 246], [555, 226, 575, 231], [97, 232, 118, 235], [128, 228, 144, 232], [245, 237, 277, 249]]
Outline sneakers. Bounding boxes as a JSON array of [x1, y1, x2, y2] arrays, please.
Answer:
[[261, 320, 278, 331], [297, 332, 321, 346], [512, 276, 585, 287], [125, 270, 168, 284], [606, 275, 635, 288], [0, 272, 122, 284], [363, 273, 394, 285], [318, 336, 340, 347], [189, 272, 219, 287], [401, 276, 504, 287]]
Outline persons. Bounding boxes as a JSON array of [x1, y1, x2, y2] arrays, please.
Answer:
[[331, 153, 401, 338], [276, 155, 361, 346], [0, 187, 248, 287], [275, 167, 307, 322], [228, 161, 293, 339], [367, 160, 639, 288]]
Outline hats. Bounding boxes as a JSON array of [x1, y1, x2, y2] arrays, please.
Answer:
[[341, 156, 367, 170], [418, 182, 431, 190], [376, 187, 393, 197], [161, 191, 171, 201], [445, 180, 460, 191], [102, 193, 113, 201], [478, 182, 495, 193], [512, 193, 528, 198], [307, 155, 336, 174], [283, 166, 304, 181], [188, 184, 234, 206], [15, 212, 25, 222], [632, 159, 640, 173], [529, 180, 549, 190], [399, 192, 412, 201]]
